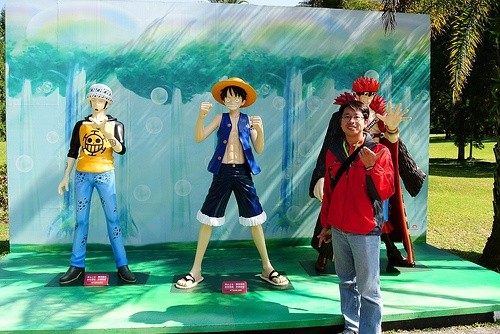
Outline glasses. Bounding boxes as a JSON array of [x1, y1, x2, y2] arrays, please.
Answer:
[[340, 115, 364, 122]]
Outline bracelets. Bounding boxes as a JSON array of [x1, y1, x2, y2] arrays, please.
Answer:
[[366, 166, 374, 170]]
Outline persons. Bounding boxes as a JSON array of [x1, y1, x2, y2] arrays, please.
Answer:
[[309, 77, 425, 274], [58, 84, 136, 284], [176, 78, 289, 289], [317, 99, 395, 334]]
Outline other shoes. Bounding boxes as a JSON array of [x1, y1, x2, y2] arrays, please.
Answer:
[[339, 329, 354, 334]]
